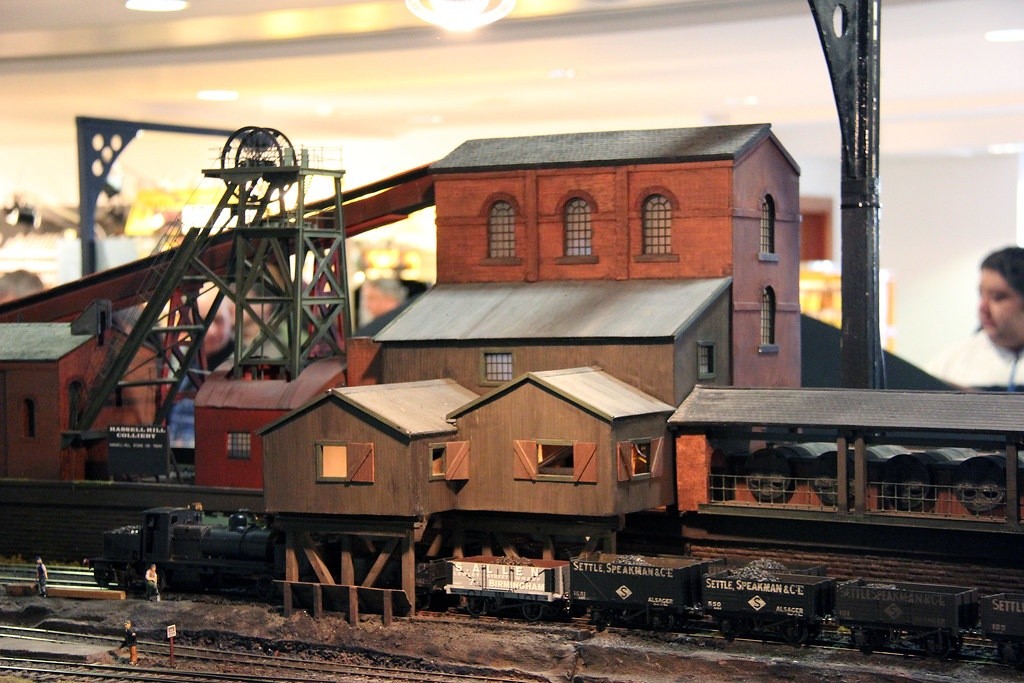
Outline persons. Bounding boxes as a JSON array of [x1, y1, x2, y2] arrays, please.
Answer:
[[974, 247, 1023, 393], [0, 270, 408, 449], [36, 558, 49, 597], [118, 621, 137, 666], [146, 564, 157, 601]]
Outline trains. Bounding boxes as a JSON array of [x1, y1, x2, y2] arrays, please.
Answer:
[[86, 500, 1024, 668]]
